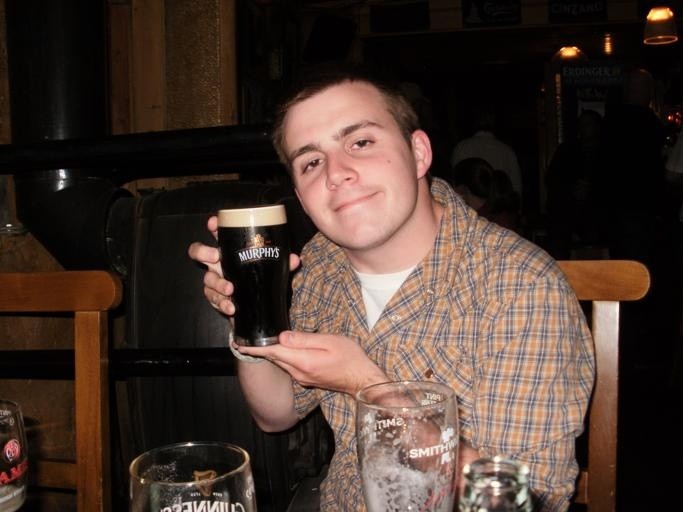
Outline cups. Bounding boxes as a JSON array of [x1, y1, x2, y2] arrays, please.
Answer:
[[354, 379, 460, 512], [128, 440, 258, 512], [0, 397, 31, 512], [216, 204, 296, 347], [459, 454, 534, 512]]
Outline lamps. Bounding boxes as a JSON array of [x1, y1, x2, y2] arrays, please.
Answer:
[[643, 6, 678, 46]]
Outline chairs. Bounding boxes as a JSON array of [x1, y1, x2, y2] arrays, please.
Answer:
[[552, 260, 653, 511], [0, 268, 123, 510]]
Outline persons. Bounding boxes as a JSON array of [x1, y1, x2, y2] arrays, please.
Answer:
[[450, 157, 523, 233], [186, 71, 599, 510], [449, 114, 524, 209]]
